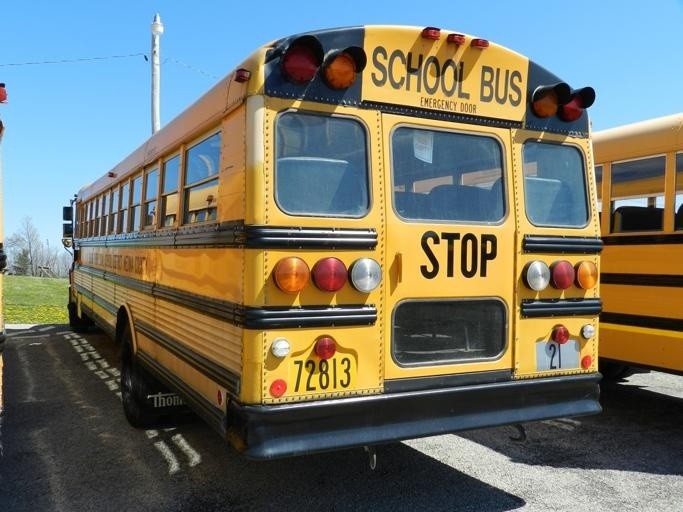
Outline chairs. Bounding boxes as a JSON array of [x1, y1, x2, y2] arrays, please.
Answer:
[[275, 154, 574, 228], [599, 201, 682, 232]]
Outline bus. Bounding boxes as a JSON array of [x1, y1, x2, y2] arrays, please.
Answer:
[[0, 79, 8, 425], [395, 108, 682, 387], [56, 23, 609, 471]]
